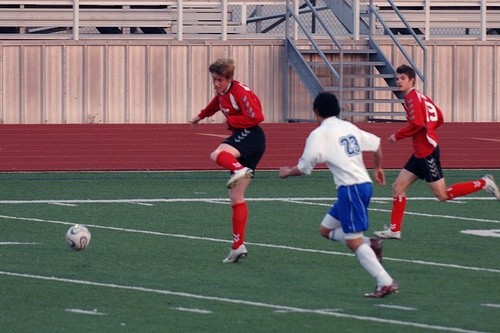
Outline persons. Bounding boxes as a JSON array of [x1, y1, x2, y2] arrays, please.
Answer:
[[279, 90, 399, 299], [374, 64, 499, 239], [189, 57, 265, 264]]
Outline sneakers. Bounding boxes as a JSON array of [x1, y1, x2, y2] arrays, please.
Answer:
[[227, 166, 253, 188], [482, 173, 500, 200], [364, 279, 399, 298], [373, 227, 401, 239], [223, 243, 248, 263], [370, 237, 383, 263]]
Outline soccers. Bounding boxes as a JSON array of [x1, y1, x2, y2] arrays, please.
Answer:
[[66, 224, 92, 250]]
